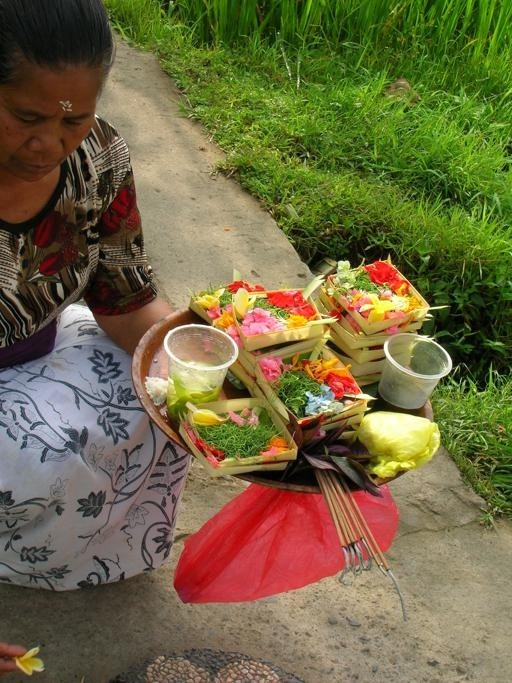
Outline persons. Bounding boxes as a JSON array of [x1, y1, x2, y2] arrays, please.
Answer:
[[1, 0, 193, 676]]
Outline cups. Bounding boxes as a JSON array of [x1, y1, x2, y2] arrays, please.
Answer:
[[375, 333, 453, 410], [163, 324, 239, 430]]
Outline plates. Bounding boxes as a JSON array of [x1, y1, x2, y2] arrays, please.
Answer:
[[133, 305, 435, 491]]
